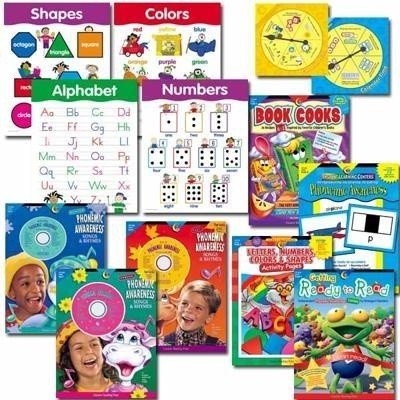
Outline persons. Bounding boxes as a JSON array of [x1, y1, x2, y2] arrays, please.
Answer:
[[162, 280, 225, 346], [56, 318, 115, 392], [5, 252, 49, 329]]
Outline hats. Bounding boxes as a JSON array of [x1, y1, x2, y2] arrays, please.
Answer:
[[56, 319, 80, 364], [5, 253, 49, 302]]
